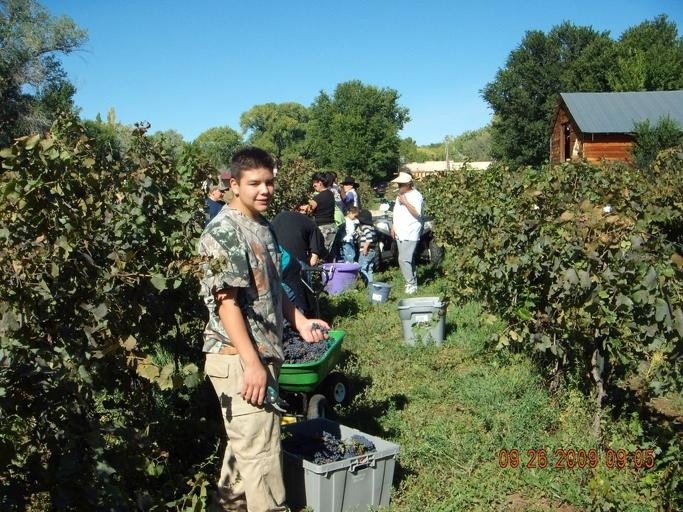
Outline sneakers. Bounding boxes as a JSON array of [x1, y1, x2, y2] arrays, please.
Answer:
[[405, 284, 415, 294]]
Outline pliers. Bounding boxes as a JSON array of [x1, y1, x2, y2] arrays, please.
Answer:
[[236, 385, 290, 413]]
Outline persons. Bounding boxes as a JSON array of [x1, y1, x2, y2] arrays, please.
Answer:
[[390, 171, 424, 294], [270, 210, 324, 314], [203, 184, 229, 229], [299, 172, 378, 284], [198, 146, 330, 512]]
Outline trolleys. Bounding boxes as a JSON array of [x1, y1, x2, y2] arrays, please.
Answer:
[[274, 265, 350, 426]]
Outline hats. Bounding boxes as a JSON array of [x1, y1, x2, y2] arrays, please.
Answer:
[[207, 181, 229, 191], [339, 177, 360, 187], [390, 172, 413, 183], [354, 209, 374, 224]]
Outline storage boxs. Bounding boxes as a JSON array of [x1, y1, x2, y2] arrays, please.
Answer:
[[278, 328, 345, 391], [321, 262, 360, 296], [276, 419, 402, 511], [398, 298, 445, 348]]
